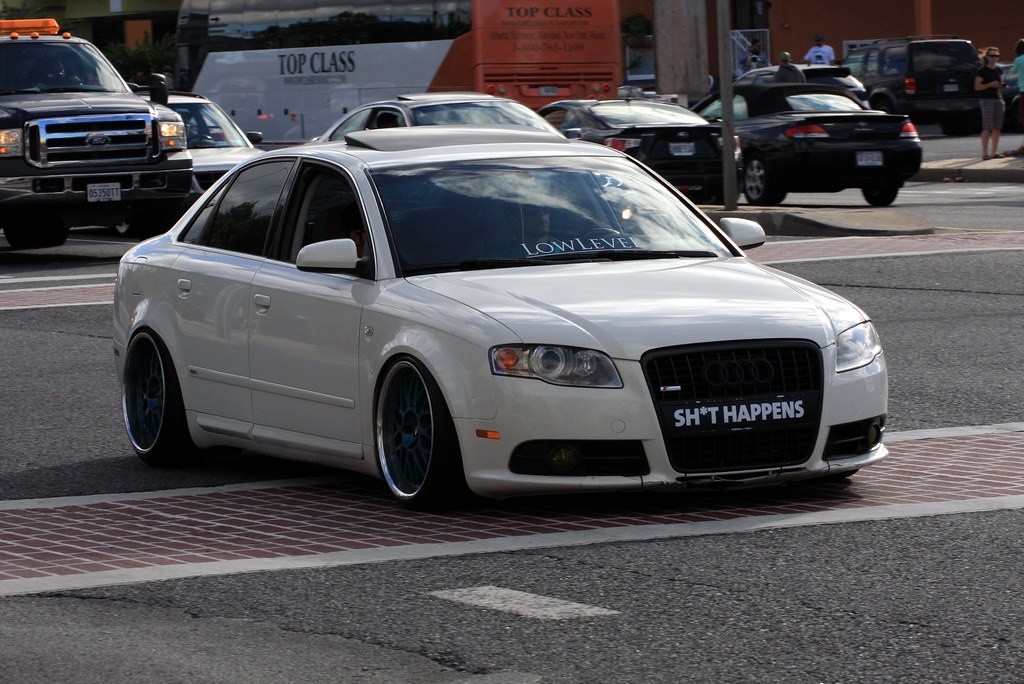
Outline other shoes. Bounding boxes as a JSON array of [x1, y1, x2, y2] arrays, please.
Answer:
[[983, 155, 990, 160], [1017, 147, 1024, 156], [992, 153, 1005, 158]]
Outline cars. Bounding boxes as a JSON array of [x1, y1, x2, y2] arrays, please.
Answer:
[[113, 123, 890, 516], [306, 91, 569, 145], [996, 62, 1022, 133], [690, 81, 924, 206], [535, 101, 725, 205], [136, 91, 263, 215]]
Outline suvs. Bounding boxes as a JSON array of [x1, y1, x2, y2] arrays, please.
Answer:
[[0, 16, 193, 252], [841, 35, 983, 138], [734, 63, 873, 113]]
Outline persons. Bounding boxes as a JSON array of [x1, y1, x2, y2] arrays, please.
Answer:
[[484, 185, 565, 257], [1007, 39, 1023, 156], [130, 63, 194, 92], [738, 38, 769, 74], [43, 59, 67, 87], [975, 47, 1005, 160], [790, 34, 835, 66], [773, 52, 807, 83]]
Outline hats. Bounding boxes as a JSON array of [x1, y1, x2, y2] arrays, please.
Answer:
[[779, 52, 791, 61], [814, 34, 824, 40]]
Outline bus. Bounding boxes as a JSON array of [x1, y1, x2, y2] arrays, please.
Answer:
[[178, 0, 619, 155]]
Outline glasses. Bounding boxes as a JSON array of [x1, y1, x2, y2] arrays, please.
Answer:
[[524, 205, 550, 214], [988, 54, 1000, 58]]
